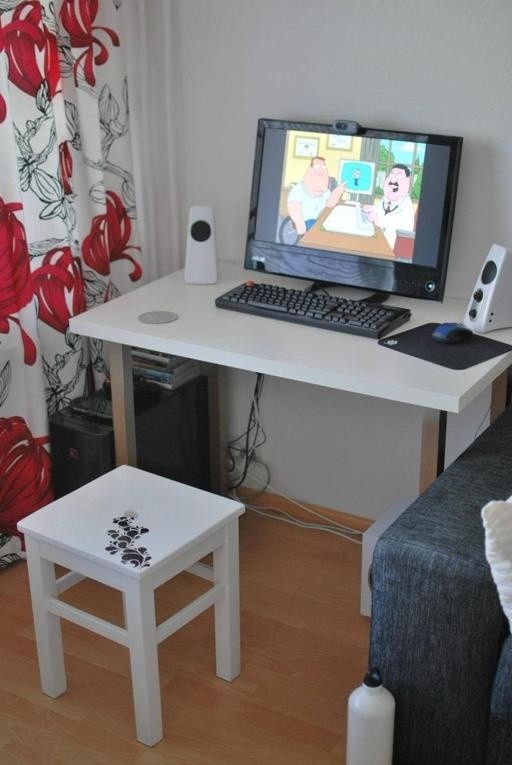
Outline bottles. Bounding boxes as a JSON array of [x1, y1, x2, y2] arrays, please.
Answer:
[[347, 672, 396, 765]]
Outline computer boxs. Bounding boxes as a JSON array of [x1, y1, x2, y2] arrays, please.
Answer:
[[50, 375, 211, 499]]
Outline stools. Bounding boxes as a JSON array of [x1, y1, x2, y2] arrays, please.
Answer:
[[17, 465, 245, 746]]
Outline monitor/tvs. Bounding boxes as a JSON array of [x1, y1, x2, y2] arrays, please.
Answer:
[[244, 118, 464, 304]]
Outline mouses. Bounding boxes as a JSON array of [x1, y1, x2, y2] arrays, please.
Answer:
[[431, 321, 471, 344]]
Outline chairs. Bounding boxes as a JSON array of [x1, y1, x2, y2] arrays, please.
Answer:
[[368, 408, 512, 765]]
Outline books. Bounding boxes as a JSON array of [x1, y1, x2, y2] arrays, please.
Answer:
[[120, 346, 207, 392]]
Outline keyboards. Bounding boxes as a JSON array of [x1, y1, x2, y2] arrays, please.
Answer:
[[216, 280, 410, 340]]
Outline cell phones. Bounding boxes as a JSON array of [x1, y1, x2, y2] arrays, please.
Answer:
[[71, 396, 112, 418]]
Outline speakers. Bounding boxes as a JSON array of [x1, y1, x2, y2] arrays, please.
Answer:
[[463, 242, 512, 333], [183, 205, 219, 285]]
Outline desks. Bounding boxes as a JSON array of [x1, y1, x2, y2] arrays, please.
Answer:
[[69, 262, 512, 497]]
